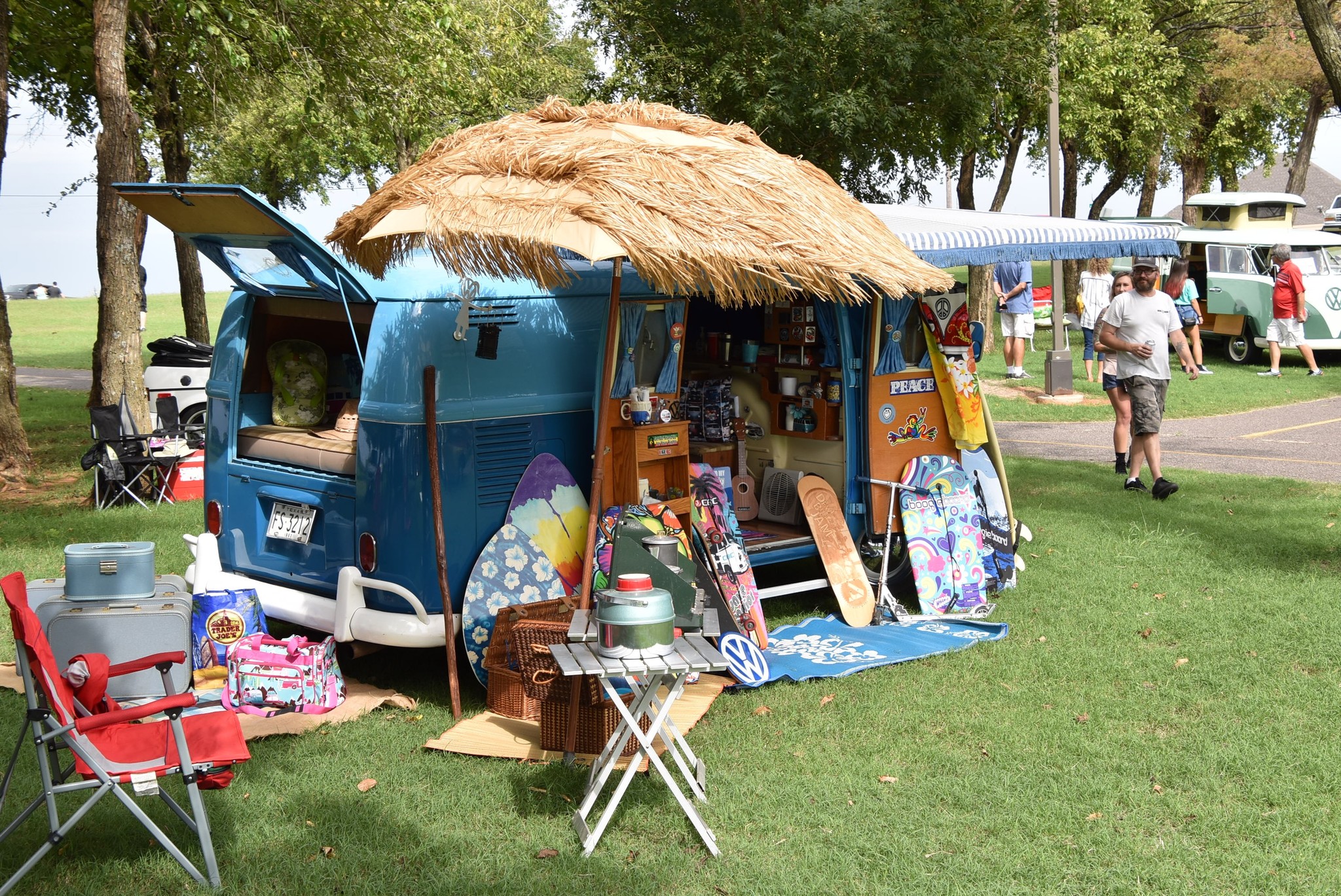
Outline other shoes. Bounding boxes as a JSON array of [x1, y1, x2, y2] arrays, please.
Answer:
[[140, 328, 145, 331]]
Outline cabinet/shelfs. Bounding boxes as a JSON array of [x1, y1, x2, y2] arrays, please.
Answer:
[[683, 295, 844, 442], [611, 420, 692, 543]]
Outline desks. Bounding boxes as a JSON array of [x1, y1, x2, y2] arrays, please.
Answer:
[[567, 608, 721, 803], [548, 635, 731, 861]]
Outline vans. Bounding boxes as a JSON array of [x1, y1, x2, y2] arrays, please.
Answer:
[[110, 99, 1190, 688], [1162, 229, 1341, 365]]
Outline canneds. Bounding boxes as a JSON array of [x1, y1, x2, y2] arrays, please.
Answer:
[[1144, 340, 1155, 352]]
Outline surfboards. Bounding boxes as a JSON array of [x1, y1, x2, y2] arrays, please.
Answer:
[[589, 501, 697, 659], [504, 452, 588, 609], [682, 461, 768, 649], [958, 436, 1018, 592], [462, 525, 570, 696], [901, 454, 991, 617]]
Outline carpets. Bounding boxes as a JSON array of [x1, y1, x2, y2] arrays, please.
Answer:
[[423, 673, 736, 773], [0, 661, 417, 741], [731, 612, 1009, 688]]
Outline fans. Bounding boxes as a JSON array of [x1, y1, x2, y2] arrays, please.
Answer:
[[758, 467, 804, 525]]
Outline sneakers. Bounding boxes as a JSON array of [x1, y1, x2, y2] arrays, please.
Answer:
[[1126, 446, 1131, 469], [1115, 458, 1128, 475], [1011, 370, 1035, 380], [1005, 373, 1012, 380], [1124, 477, 1151, 493], [1181, 365, 1186, 372], [1152, 479, 1179, 499], [1196, 364, 1214, 374], [1306, 369, 1324, 376], [1257, 369, 1282, 377]]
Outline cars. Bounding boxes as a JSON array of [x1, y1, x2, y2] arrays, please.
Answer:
[[1098, 217, 1189, 286], [4, 284, 56, 301], [1317, 195, 1341, 232], [143, 334, 215, 448]]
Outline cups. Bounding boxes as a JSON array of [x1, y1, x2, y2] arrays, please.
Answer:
[[620, 401, 652, 425], [785, 406, 795, 431], [742, 343, 758, 370], [778, 377, 798, 396], [826, 379, 841, 403], [705, 332, 718, 359]]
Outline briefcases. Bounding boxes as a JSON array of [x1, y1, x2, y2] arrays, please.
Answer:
[[33, 590, 192, 638], [63, 541, 156, 600], [26, 574, 187, 614], [47, 604, 192, 701]]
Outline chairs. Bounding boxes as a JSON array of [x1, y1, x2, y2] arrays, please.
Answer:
[[1030, 285, 1072, 353], [156, 396, 205, 450], [90, 405, 184, 512], [0, 570, 252, 896]]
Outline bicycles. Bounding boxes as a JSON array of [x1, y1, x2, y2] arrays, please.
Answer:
[[854, 474, 996, 627]]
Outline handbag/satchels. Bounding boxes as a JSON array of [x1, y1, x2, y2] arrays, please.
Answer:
[[193, 588, 269, 689], [1076, 295, 1084, 316], [221, 632, 347, 717], [1184, 317, 1196, 325]]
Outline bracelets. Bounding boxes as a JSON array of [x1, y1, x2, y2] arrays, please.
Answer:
[[1198, 315, 1202, 317]]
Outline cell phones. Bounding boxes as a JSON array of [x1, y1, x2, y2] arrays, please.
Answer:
[[998, 304, 1008, 309]]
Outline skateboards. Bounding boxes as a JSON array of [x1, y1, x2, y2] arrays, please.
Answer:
[[798, 471, 877, 629], [685, 498, 768, 650]]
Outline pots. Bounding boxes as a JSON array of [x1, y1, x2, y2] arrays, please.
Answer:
[[641, 534, 679, 567], [665, 565, 683, 578]]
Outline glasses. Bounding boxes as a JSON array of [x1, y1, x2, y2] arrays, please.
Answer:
[[1133, 268, 1157, 275], [1270, 253, 1275, 257]]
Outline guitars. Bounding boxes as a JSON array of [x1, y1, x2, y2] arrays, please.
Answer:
[[731, 418, 760, 522]]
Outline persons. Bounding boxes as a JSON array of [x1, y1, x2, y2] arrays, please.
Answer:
[[47, 282, 65, 298], [138, 264, 148, 331], [1163, 258, 1214, 374], [1099, 255, 1201, 500], [992, 260, 1037, 381], [34, 283, 48, 300], [1093, 271, 1135, 475], [1077, 257, 1115, 383], [1256, 243, 1324, 379]]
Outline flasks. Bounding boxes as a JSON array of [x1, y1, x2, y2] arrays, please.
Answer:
[[593, 573, 676, 660], [718, 333, 733, 366]]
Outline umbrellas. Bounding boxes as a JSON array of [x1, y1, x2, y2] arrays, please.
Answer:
[[327, 94, 954, 642]]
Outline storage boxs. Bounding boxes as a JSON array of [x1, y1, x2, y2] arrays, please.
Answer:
[[483, 595, 580, 720], [511, 619, 651, 756], [156, 450, 204, 502]]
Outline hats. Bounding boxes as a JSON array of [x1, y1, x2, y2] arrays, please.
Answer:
[[1132, 257, 1160, 268], [153, 439, 200, 457], [308, 399, 361, 441]]
